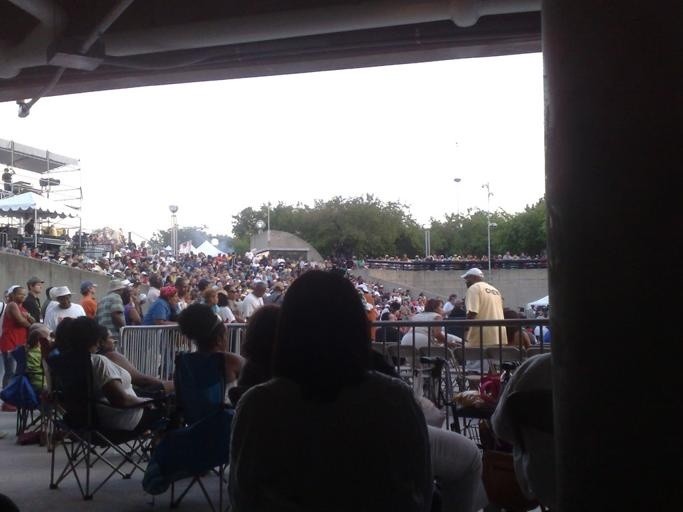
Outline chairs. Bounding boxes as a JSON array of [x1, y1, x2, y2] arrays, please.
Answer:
[[45, 350, 156, 500], [3, 348, 46, 441], [155, 395, 231, 510], [231, 335, 558, 511]]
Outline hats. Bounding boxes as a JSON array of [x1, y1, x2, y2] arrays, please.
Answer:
[[49, 284, 75, 301], [459, 267, 484, 281], [26, 275, 45, 285], [106, 258, 287, 299], [80, 279, 98, 294]]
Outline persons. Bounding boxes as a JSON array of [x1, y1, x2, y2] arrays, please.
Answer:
[[2, 168, 15, 191], [1, 219, 558, 511]]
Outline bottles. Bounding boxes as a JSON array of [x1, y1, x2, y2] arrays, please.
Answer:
[[500, 368, 513, 396], [477, 372, 500, 405]]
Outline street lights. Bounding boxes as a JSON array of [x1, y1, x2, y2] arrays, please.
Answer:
[[254, 217, 263, 234], [423, 222, 433, 257], [453, 168, 493, 281], [168, 204, 181, 259]]
